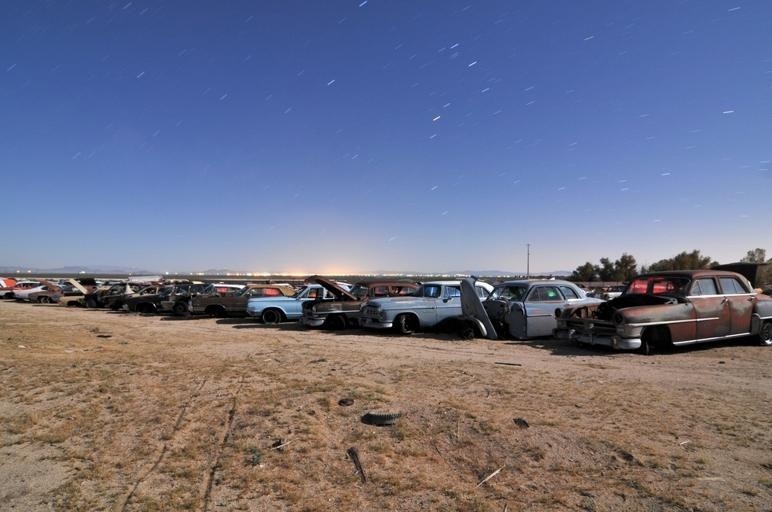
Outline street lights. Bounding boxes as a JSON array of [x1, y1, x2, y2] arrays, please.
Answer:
[[524, 242, 532, 278]]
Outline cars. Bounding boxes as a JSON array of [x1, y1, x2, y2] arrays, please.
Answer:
[[0, 277, 420, 331], [358, 280, 512, 337], [552, 264, 772, 354], [458, 278, 609, 341]]
